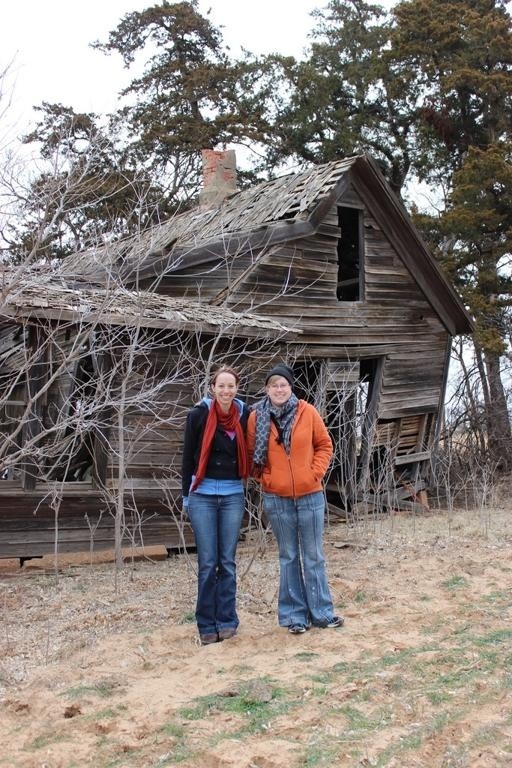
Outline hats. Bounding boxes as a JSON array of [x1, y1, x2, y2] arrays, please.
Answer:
[[265, 365, 294, 390]]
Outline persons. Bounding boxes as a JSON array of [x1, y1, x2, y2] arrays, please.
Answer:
[[179, 370, 253, 647], [245, 362, 343, 634]]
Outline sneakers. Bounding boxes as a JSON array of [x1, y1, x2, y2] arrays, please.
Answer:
[[218, 627, 236, 641], [326, 616, 344, 628], [288, 622, 307, 633], [200, 631, 217, 644]]
